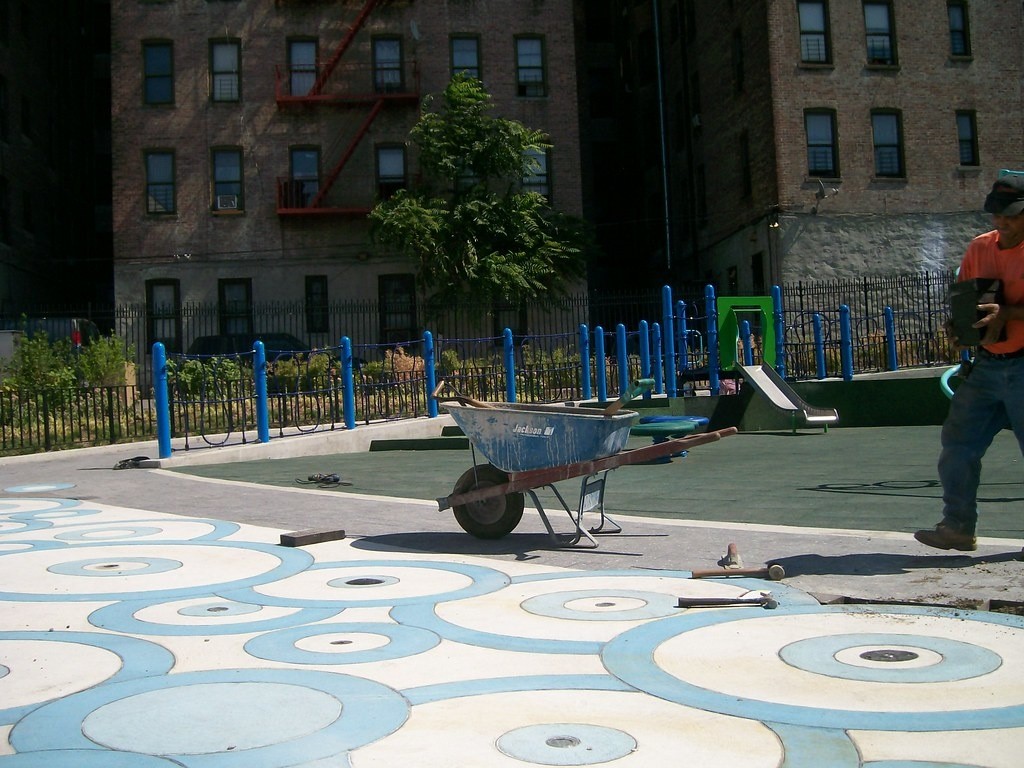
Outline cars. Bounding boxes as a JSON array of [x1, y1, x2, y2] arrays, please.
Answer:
[[4, 317, 102, 351], [186, 335, 369, 379]]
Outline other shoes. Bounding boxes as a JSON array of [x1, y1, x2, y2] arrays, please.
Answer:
[[915, 522, 977, 551]]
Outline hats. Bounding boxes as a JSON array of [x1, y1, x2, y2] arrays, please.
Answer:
[[984, 175, 1024, 212]]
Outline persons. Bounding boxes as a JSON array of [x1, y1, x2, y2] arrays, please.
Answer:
[[913, 175, 1024, 551]]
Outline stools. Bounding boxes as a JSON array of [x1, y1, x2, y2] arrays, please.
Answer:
[[639, 415, 709, 458], [630, 423, 694, 463]]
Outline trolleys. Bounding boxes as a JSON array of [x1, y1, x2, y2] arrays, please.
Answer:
[[437, 391, 737, 552]]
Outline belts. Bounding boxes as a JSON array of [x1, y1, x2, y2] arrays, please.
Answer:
[[980, 345, 1024, 359]]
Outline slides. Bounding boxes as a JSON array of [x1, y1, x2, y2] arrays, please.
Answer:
[[736, 359, 840, 425]]
